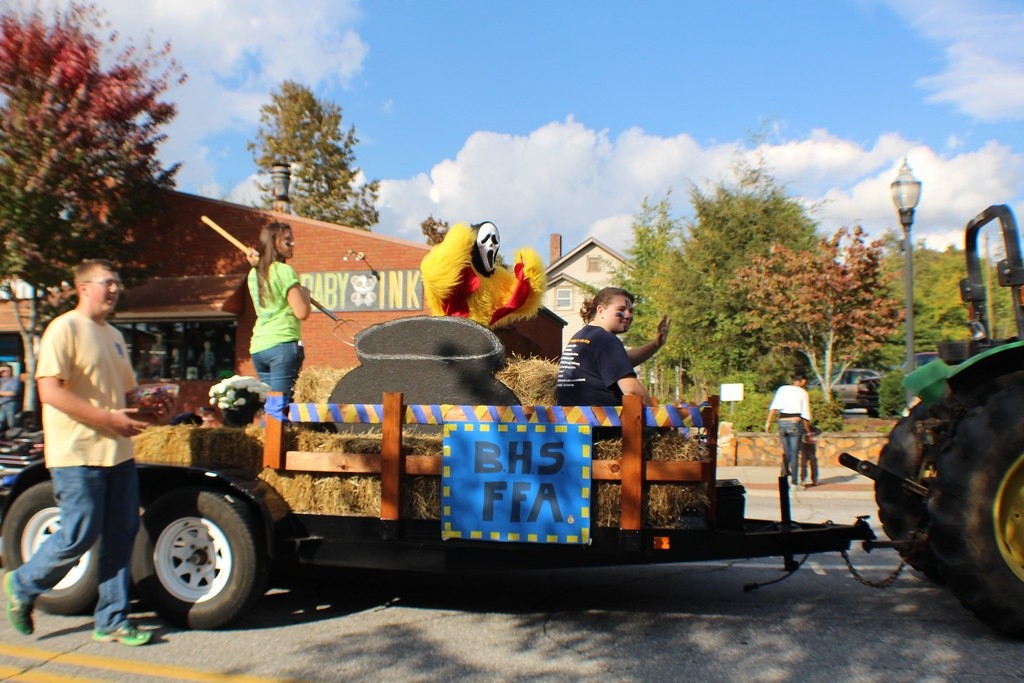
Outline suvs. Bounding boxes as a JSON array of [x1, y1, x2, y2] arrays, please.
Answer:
[[854, 349, 939, 417]]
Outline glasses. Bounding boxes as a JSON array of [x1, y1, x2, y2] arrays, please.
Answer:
[[84, 280, 123, 291]]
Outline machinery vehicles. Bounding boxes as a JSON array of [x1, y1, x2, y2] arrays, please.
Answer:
[[837, 201, 1024, 646]]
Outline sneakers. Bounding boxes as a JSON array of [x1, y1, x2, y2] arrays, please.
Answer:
[[93, 623, 154, 646], [4, 571, 34, 635]]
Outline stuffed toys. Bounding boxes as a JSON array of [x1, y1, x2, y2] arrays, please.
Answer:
[[420, 220, 548, 329]]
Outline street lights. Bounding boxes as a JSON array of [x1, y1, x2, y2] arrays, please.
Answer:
[[888, 156, 926, 406]]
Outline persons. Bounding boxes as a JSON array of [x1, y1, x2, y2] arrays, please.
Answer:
[[246, 223, 311, 403], [555, 286, 671, 436], [766, 372, 821, 489], [0, 365, 20, 428], [4, 259, 173, 647]]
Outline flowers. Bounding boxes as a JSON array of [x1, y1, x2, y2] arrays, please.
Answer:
[[208, 376, 270, 412]]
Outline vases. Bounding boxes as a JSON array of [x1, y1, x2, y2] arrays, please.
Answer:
[[224, 393, 259, 428]]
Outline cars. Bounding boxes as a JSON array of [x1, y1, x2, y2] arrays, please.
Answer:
[[802, 368, 887, 410]]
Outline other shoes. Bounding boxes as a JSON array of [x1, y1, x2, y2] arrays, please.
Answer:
[[801, 481, 805, 485], [791, 484, 805, 491], [813, 482, 817, 486]]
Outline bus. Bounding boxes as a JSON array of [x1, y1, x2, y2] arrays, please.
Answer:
[[110, 302, 248, 433]]
